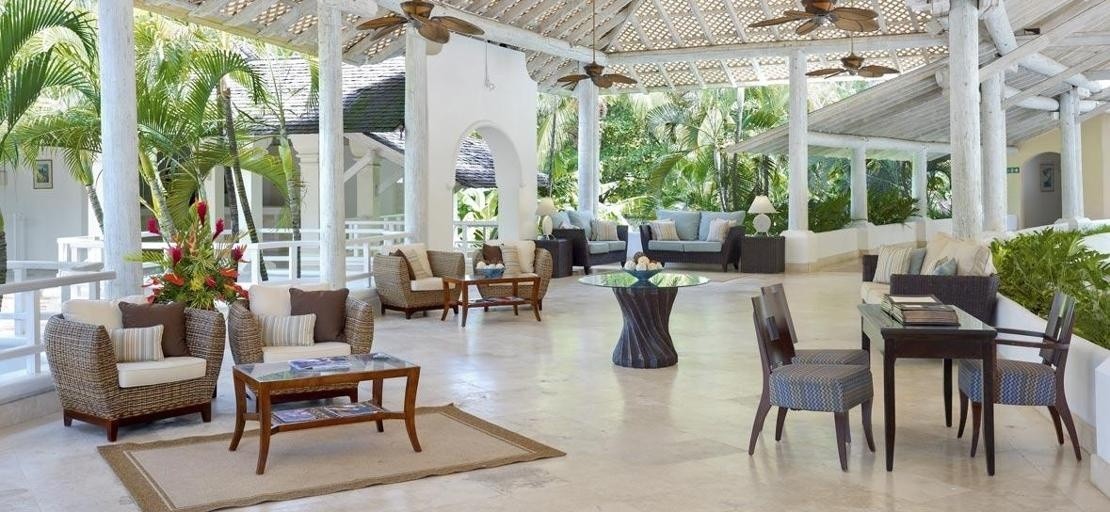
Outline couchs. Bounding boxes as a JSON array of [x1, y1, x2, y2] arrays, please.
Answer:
[[551, 210, 628, 275], [639, 209, 746, 272]]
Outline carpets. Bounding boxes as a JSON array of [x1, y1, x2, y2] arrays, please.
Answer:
[[97, 403, 566, 512]]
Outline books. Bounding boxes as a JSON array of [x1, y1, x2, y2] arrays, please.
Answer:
[[273, 408, 322, 425], [288, 356, 352, 372], [880, 292, 962, 326], [320, 403, 380, 417]]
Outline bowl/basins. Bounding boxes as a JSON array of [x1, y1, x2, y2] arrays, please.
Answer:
[[474, 268, 507, 277]]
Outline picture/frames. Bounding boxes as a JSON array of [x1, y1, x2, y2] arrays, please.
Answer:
[[33, 159, 53, 190], [1039, 163, 1054, 192]]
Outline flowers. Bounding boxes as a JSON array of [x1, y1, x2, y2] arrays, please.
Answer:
[[123, 202, 254, 309]]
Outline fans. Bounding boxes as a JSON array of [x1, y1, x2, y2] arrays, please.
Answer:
[[557, 0, 638, 91], [355, 0, 484, 43], [747, 0, 880, 36], [804, 1, 900, 79]]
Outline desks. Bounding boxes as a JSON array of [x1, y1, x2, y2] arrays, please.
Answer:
[[578, 271, 712, 368]]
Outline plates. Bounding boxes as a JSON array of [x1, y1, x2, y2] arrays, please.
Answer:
[[623, 269, 662, 279], [629, 280, 658, 288]]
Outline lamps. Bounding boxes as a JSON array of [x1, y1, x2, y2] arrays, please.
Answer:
[[535, 198, 554, 240], [748, 195, 777, 237]]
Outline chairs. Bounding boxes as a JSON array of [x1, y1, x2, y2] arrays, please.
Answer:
[[472, 239, 553, 312], [372, 244, 465, 319], [43, 294, 226, 441], [228, 282, 374, 412]]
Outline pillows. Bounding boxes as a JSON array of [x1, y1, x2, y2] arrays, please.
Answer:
[[552, 210, 618, 242], [389, 249, 417, 280], [62, 299, 189, 362], [482, 243, 504, 266], [484, 239, 536, 273], [396, 247, 430, 279], [249, 283, 349, 346], [649, 209, 746, 241]]
